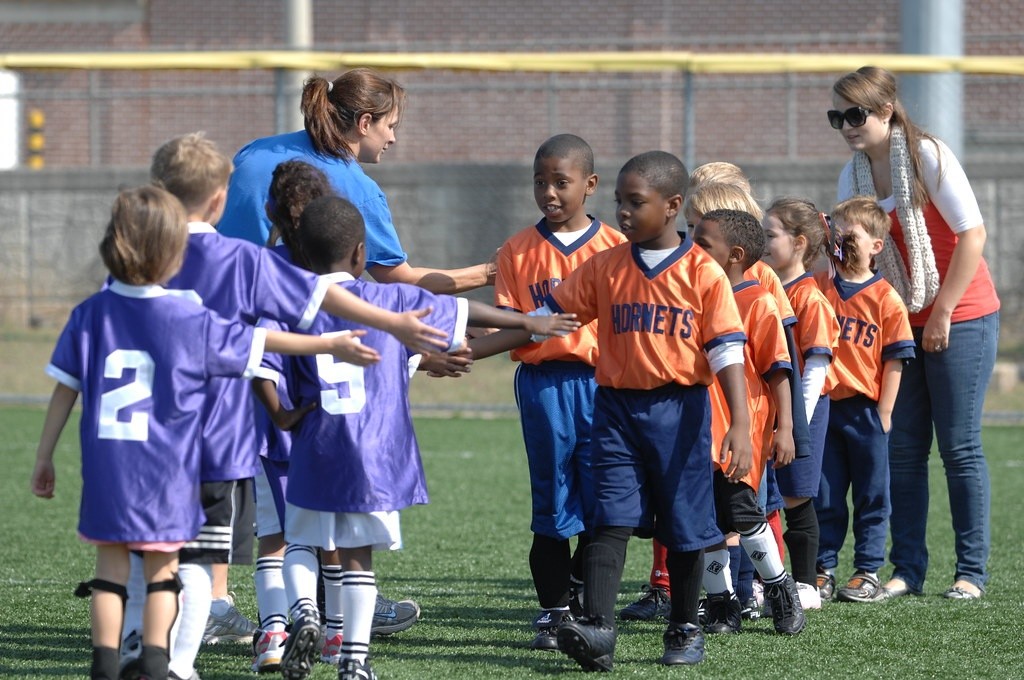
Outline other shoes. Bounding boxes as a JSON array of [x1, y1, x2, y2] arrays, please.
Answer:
[[872, 586, 913, 601], [944, 585, 978, 601]]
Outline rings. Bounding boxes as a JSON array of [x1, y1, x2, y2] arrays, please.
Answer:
[[935, 345, 941, 349]]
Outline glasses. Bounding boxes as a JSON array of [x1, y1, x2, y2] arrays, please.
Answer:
[[828, 106, 872, 130]]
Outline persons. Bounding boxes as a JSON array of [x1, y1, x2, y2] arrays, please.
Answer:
[[31, 186, 381, 680], [826, 65, 1001, 598], [118, 125, 449, 680], [214, 66, 503, 636], [254, 159, 581, 680], [426, 132, 916, 672]]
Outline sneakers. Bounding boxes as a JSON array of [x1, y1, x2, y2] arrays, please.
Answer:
[[337, 656, 376, 680], [114, 629, 144, 680], [663, 626, 703, 665], [621, 583, 672, 620], [813, 570, 836, 601], [370, 594, 421, 635], [556, 620, 615, 671], [562, 591, 590, 626], [836, 572, 880, 604], [280, 610, 320, 680], [199, 591, 258, 644], [530, 611, 571, 648], [318, 634, 344, 664], [253, 628, 293, 671], [697, 578, 821, 636]]
